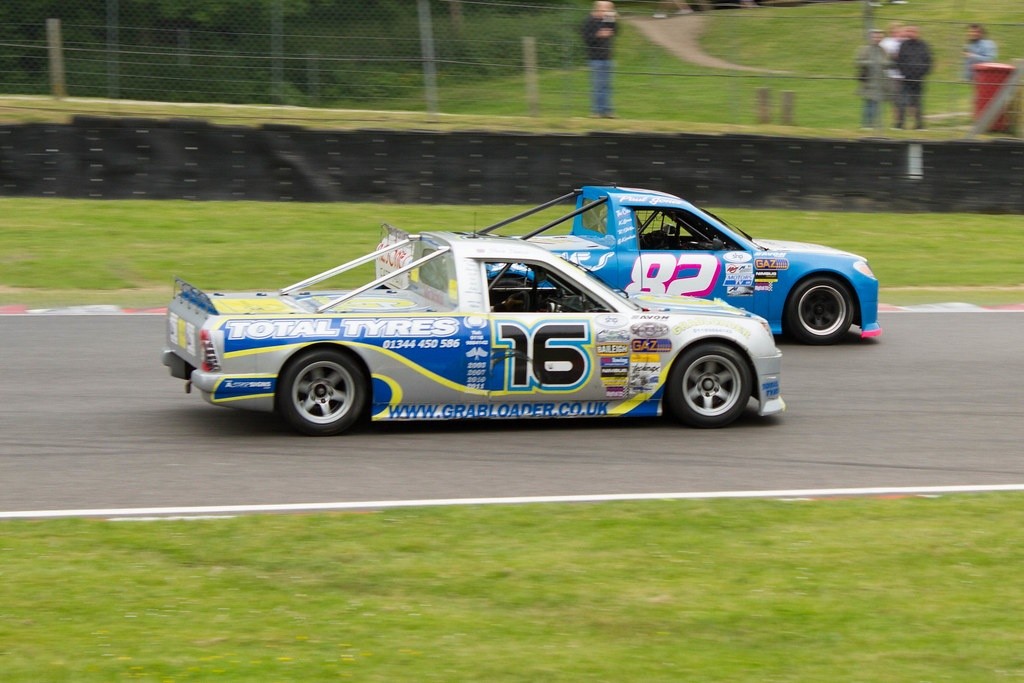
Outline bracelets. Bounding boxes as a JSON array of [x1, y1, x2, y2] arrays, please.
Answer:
[[494, 301, 506, 312]]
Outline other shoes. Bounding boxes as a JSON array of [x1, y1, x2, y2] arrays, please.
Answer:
[[912, 121, 922, 130], [892, 122, 903, 129]]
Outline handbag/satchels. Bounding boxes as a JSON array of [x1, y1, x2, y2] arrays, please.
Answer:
[[853, 65, 869, 81]]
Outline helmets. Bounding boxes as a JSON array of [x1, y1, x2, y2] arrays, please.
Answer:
[[419, 255, 447, 290]]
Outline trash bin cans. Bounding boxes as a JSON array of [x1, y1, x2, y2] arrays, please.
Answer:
[[968, 62, 1017, 130]]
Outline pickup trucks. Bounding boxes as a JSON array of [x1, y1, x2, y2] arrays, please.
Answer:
[[483, 186, 881, 346], [162, 231, 788, 436]]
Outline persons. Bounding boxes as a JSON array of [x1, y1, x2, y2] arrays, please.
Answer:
[[580, 0, 624, 118], [490, 292, 525, 312], [961, 22, 998, 80], [852, 21, 930, 130]]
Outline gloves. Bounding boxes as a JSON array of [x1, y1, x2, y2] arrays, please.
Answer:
[[501, 294, 522, 310]]
